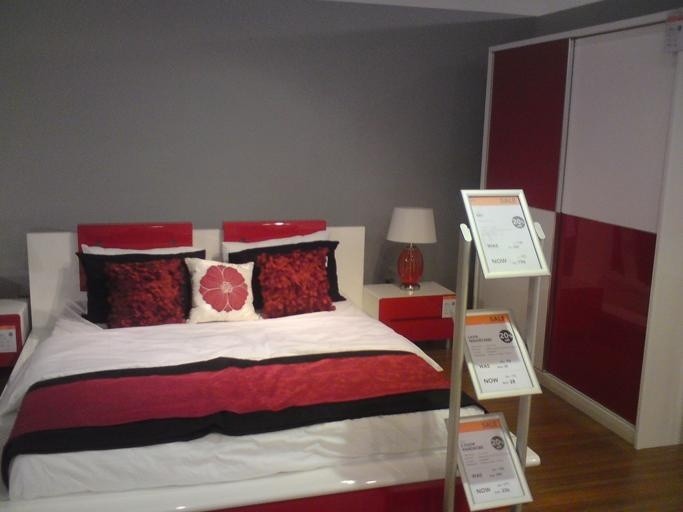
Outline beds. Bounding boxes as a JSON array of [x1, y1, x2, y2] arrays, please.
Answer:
[[0, 225, 540, 512]]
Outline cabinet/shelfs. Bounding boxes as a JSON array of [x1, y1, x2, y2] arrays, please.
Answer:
[[472, 6, 683, 449]]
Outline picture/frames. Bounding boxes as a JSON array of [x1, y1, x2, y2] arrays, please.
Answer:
[[462, 187, 549, 278], [442, 410, 533, 512], [454, 308, 543, 399]]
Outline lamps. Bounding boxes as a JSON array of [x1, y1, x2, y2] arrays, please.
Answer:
[[386, 207, 437, 291]]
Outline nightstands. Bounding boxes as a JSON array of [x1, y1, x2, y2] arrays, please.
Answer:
[[364, 280, 457, 360], [0, 297, 30, 369]]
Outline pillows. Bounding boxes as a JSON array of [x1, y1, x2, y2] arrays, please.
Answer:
[[73, 220, 346, 328]]
